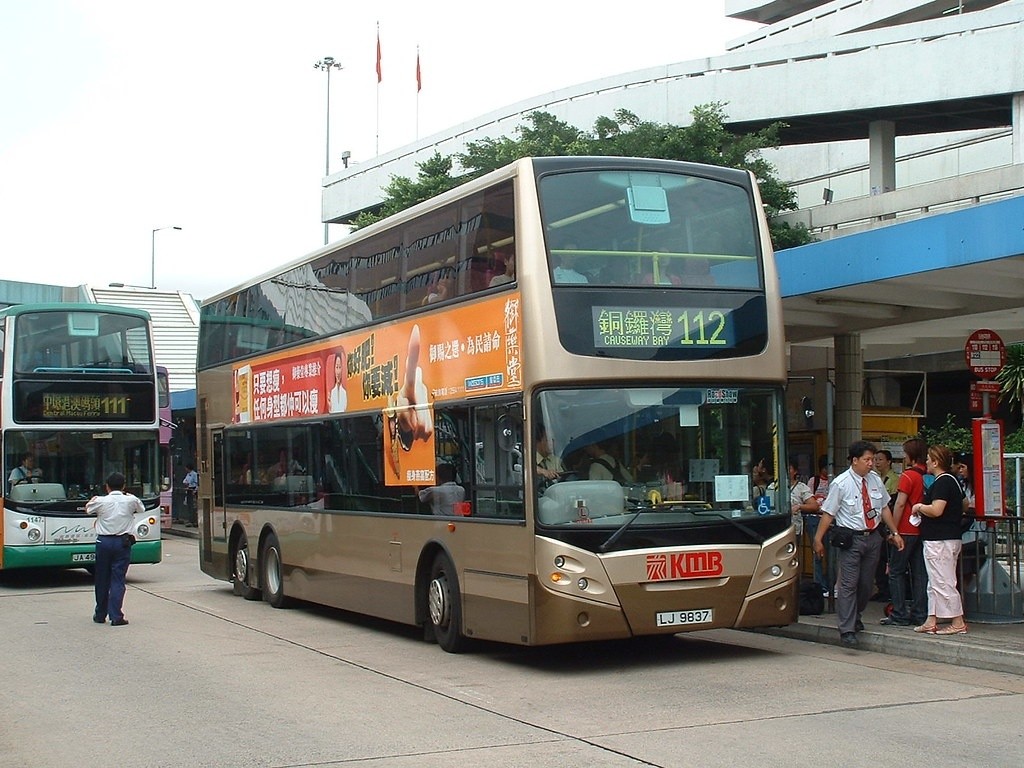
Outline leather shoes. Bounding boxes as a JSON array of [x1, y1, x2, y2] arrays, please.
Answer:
[[841, 632, 860, 646], [855, 619, 864, 632]]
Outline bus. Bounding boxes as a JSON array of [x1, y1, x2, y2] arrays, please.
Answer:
[[196, 155, 816, 654], [79, 362, 173, 530], [0, 302, 180, 570]]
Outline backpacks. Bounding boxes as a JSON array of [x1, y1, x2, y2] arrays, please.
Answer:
[[594, 458, 627, 486]]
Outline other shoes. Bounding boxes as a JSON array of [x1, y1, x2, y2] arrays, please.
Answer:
[[822, 590, 829, 597], [111, 619, 128, 626], [913, 624, 936, 634], [936, 624, 969, 635], [907, 616, 926, 625], [879, 617, 909, 625], [834, 585, 838, 598], [93, 614, 106, 623]]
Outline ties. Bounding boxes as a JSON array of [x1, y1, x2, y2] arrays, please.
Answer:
[[862, 478, 875, 529], [542, 458, 553, 487]]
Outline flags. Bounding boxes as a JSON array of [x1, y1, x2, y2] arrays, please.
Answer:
[[376, 32, 381, 82], [417, 53, 421, 93]]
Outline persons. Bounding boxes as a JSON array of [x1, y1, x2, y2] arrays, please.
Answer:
[[85, 472, 145, 626], [412, 463, 465, 516], [182, 464, 200, 527], [9, 453, 42, 492], [427, 268, 456, 303], [516, 423, 568, 498], [584, 428, 633, 486], [268, 448, 303, 481], [236, 451, 267, 485], [806, 438, 997, 647], [554, 239, 588, 283], [488, 251, 515, 288], [327, 353, 348, 414], [745, 457, 820, 547], [647, 418, 686, 484], [641, 244, 681, 285]]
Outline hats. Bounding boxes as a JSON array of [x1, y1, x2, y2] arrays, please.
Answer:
[[959, 452, 974, 465]]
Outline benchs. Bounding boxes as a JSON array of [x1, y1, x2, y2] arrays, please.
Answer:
[[370, 257, 507, 320]]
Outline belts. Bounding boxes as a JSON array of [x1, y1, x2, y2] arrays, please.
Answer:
[[854, 528, 877, 536]]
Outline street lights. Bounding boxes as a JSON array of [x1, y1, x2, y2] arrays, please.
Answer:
[[152, 226, 182, 288], [313, 57, 343, 245]]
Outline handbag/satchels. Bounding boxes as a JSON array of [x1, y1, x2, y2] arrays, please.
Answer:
[[829, 527, 853, 549]]
